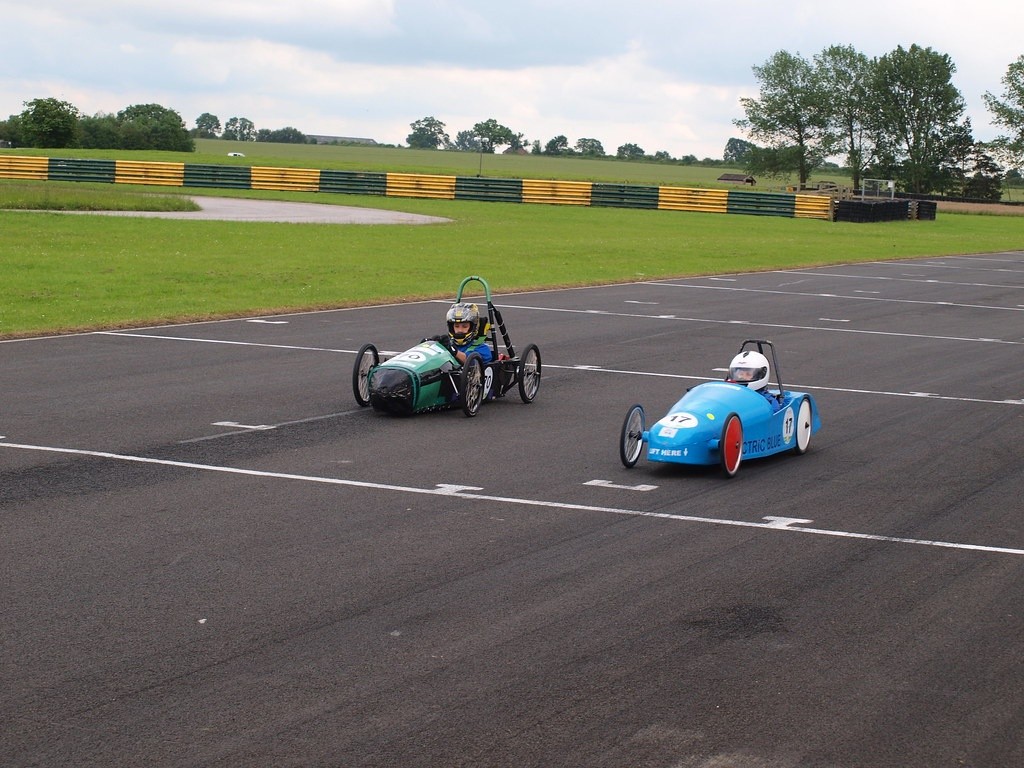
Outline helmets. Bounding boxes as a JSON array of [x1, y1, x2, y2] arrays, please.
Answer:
[[446, 303, 479, 346], [728, 351, 770, 391]]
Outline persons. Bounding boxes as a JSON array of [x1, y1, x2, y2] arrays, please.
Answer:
[[418, 303, 494, 372], [722, 349, 783, 416]]
